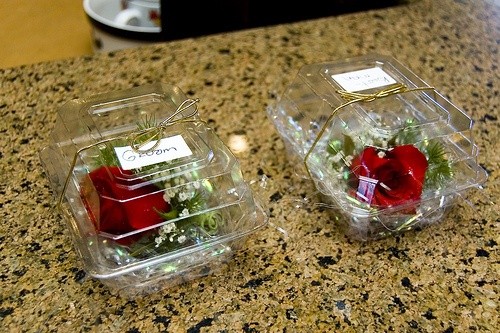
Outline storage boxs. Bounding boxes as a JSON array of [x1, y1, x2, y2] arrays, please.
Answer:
[[267, 54, 489, 243], [40, 84, 268, 298]]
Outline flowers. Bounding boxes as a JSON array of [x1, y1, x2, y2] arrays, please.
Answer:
[[78, 116, 225, 256], [326, 119, 461, 214]]
